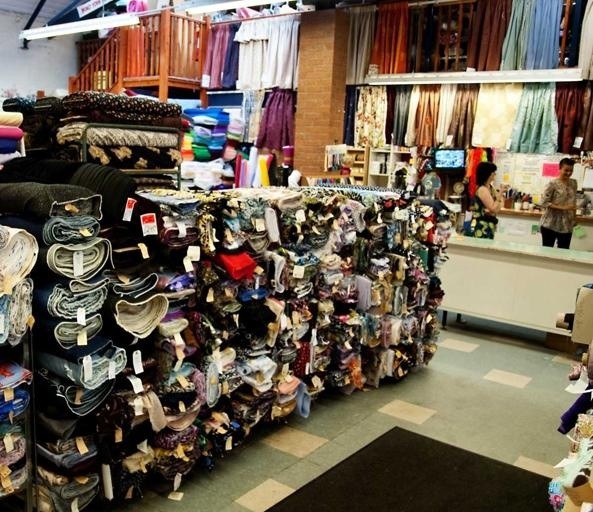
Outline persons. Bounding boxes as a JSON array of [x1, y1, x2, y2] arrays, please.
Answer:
[[539, 157, 580, 249], [471, 161, 509, 239]]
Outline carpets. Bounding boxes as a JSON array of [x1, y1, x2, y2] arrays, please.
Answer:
[[264, 426, 554, 512]]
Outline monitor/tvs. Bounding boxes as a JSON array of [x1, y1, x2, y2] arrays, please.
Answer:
[[434, 150, 465, 169]]
